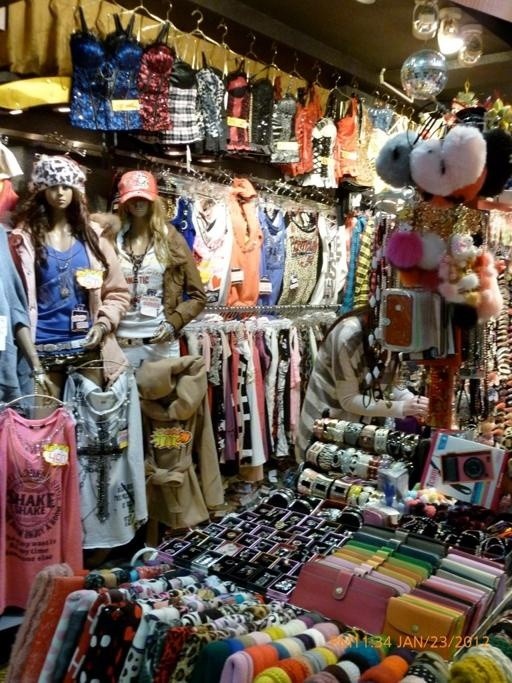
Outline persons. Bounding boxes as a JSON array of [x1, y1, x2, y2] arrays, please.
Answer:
[[15, 154, 130, 422], [296, 291, 429, 470], [91, 171, 206, 421], [1, 139, 60, 420]]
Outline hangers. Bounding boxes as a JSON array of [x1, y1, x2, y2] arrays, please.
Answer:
[[107, 2, 327, 109], [328, 70, 416, 122], [184, 302, 347, 331], [68, 339, 139, 381], [147, 159, 340, 211], [0, 364, 67, 410]]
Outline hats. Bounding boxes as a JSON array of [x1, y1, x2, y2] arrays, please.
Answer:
[[28, 157, 85, 193], [118, 170, 156, 204]]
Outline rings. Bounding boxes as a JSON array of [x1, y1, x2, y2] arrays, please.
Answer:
[[417, 399, 420, 403], [418, 395, 420, 398]]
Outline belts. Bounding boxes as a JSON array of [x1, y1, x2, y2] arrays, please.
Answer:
[[116, 336, 158, 346], [35, 337, 91, 352]]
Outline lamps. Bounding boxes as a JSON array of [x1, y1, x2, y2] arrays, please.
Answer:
[[411, 0, 485, 67]]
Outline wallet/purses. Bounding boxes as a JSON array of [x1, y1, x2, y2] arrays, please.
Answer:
[[290, 523, 506, 662]]
